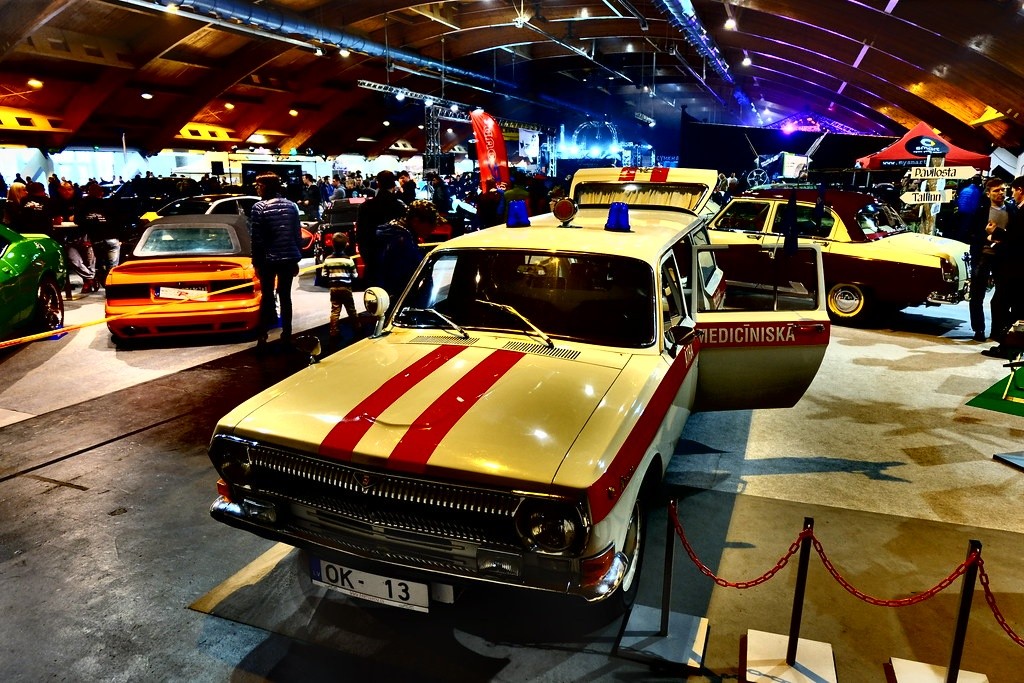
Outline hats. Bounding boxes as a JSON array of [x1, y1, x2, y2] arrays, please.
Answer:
[[532, 174, 547, 180]]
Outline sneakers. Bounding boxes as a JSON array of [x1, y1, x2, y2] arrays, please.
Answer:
[[973, 331, 986, 342]]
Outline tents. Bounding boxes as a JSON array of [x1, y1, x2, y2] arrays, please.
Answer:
[[851, 120, 991, 187]]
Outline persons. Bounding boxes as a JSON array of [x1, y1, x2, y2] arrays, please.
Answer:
[[130, 168, 452, 228], [366, 200, 438, 325], [359, 171, 409, 286], [249, 171, 303, 359], [1, 171, 127, 296], [718, 172, 738, 205], [475, 173, 552, 228], [320, 232, 366, 340], [905, 179, 919, 192], [959, 175, 1024, 361]]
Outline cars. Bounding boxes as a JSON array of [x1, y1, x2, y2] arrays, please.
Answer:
[[105, 213, 263, 342], [0, 224, 67, 335], [137, 193, 262, 217], [707, 188, 973, 326], [314, 197, 452, 283], [208, 168, 833, 617]]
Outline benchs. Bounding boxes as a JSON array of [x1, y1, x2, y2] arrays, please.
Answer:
[[474, 282, 651, 342]]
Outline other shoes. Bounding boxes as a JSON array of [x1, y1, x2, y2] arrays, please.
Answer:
[[81, 279, 100, 293], [256, 337, 266, 354], [280, 333, 289, 349]]
